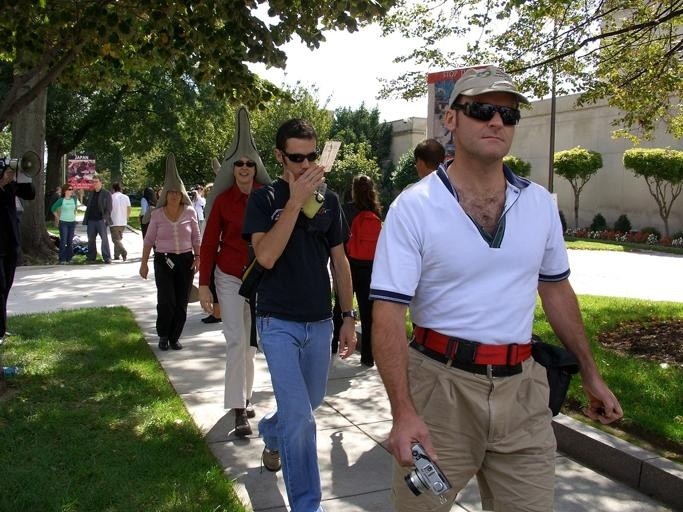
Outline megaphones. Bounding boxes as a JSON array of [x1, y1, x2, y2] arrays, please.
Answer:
[[8, 151, 42, 177]]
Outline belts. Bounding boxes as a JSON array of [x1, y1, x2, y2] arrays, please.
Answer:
[[410, 340, 522, 377]]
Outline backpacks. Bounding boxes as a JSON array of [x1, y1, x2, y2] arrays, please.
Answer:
[[345, 211, 381, 261]]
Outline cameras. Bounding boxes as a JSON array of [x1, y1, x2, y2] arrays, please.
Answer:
[[404, 444, 451, 498]]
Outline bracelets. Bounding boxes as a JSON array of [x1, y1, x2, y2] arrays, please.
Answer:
[[341, 309, 359, 324]]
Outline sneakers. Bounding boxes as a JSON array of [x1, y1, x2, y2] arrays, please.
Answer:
[[235, 408, 255, 436], [263, 445, 283, 473]]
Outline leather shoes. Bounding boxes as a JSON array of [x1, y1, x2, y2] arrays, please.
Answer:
[[159, 337, 169, 350], [170, 337, 182, 350], [202, 315, 222, 323]]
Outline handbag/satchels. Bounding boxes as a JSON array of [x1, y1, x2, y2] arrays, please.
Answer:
[[529, 338, 580, 417]]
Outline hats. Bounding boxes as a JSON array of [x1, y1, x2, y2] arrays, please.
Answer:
[[448, 66, 529, 110]]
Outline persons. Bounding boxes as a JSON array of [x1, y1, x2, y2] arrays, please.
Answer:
[[140, 182, 202, 351], [368, 67, 625, 511], [51, 185, 77, 265], [332, 174, 383, 367], [140, 182, 205, 258], [200, 184, 222, 324], [405, 139, 446, 190], [82, 175, 112, 264], [235, 117, 365, 511], [109, 183, 132, 261], [199, 156, 265, 438], [0, 153, 35, 346]]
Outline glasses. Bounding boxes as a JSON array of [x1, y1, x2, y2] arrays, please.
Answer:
[[234, 160, 257, 167], [281, 148, 320, 163], [452, 102, 521, 126]]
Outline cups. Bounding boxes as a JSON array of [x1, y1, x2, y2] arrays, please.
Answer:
[[301, 189, 324, 220]]
